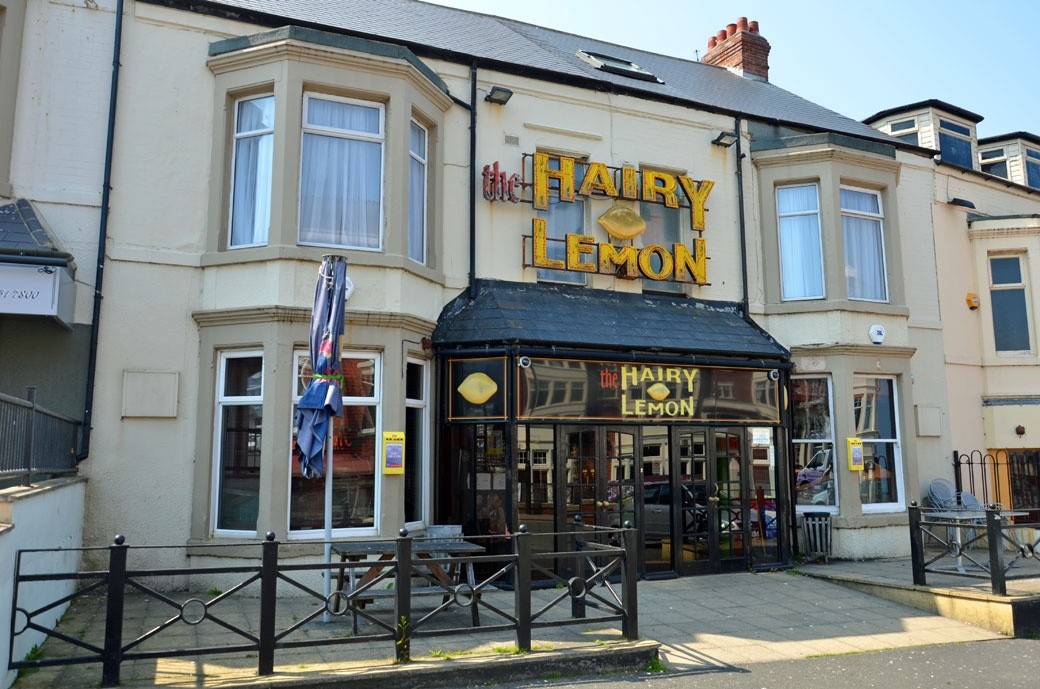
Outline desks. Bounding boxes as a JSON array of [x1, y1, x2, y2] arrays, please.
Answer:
[[332, 542, 486, 636], [920, 511, 1028, 574]]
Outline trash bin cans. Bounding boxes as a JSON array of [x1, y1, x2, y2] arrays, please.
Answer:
[[424, 525, 463, 584]]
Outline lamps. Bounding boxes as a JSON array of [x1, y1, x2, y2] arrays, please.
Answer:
[[946, 197, 976, 210], [711, 131, 739, 148], [484, 86, 514, 106]]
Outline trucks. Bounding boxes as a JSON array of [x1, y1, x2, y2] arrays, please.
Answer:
[[794, 446, 836, 500]]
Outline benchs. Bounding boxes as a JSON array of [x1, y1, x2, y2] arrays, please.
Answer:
[[322, 569, 461, 612], [336, 584, 498, 636]]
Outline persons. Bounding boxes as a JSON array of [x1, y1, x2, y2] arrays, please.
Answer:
[[488, 490, 508, 543]]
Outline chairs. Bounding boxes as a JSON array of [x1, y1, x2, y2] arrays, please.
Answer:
[[926, 479, 1021, 554]]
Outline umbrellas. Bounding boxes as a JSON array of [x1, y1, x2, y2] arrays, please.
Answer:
[[295, 254, 347, 623]]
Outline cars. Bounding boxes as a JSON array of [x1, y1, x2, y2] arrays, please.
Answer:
[[607, 479, 742, 545]]
